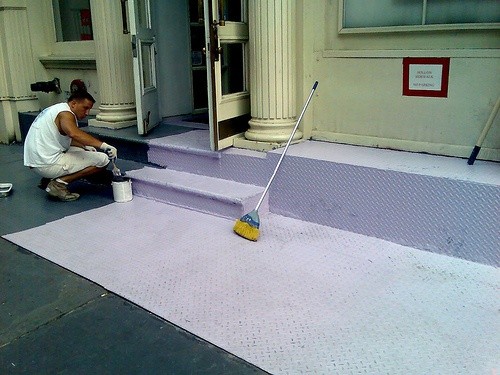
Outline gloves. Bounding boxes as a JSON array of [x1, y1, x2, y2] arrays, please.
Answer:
[[83, 145, 96, 152], [100, 142, 118, 160]]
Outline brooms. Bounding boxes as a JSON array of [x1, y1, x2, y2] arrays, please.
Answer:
[[232, 80, 319, 243]]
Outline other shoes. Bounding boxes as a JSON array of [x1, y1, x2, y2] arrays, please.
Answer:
[[45, 180, 81, 202]]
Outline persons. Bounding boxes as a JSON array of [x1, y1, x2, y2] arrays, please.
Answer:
[[23, 89, 118, 201]]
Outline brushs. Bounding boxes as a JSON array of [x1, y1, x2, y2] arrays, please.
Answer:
[[112, 160, 121, 177]]
